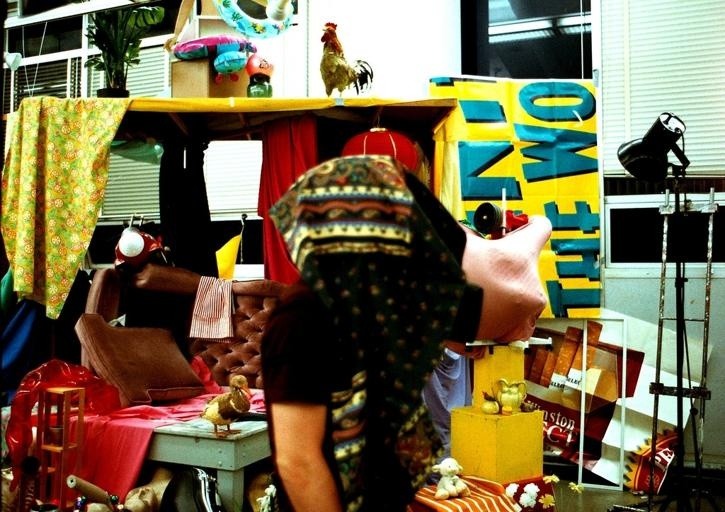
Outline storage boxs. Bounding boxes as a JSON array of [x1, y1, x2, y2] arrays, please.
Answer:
[[171, 58, 250, 98]]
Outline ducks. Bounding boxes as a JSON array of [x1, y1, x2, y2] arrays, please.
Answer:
[[200, 374, 252, 438]]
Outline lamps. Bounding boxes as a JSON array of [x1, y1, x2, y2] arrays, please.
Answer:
[[618, 112, 704, 512], [119, 214, 145, 258]]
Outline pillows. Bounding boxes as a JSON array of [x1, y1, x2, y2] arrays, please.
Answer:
[[75, 313, 204, 407]]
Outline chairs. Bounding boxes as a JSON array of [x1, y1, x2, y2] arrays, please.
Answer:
[[82, 263, 282, 387]]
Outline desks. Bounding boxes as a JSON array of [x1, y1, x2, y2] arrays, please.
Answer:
[[150, 417, 273, 512]]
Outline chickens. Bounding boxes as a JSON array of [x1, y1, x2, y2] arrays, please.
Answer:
[[320, 23, 373, 98]]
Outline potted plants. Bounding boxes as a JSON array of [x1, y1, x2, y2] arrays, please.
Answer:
[[84, 6, 165, 98]]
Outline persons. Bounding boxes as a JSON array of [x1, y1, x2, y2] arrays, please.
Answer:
[[260, 273, 416, 512]]
